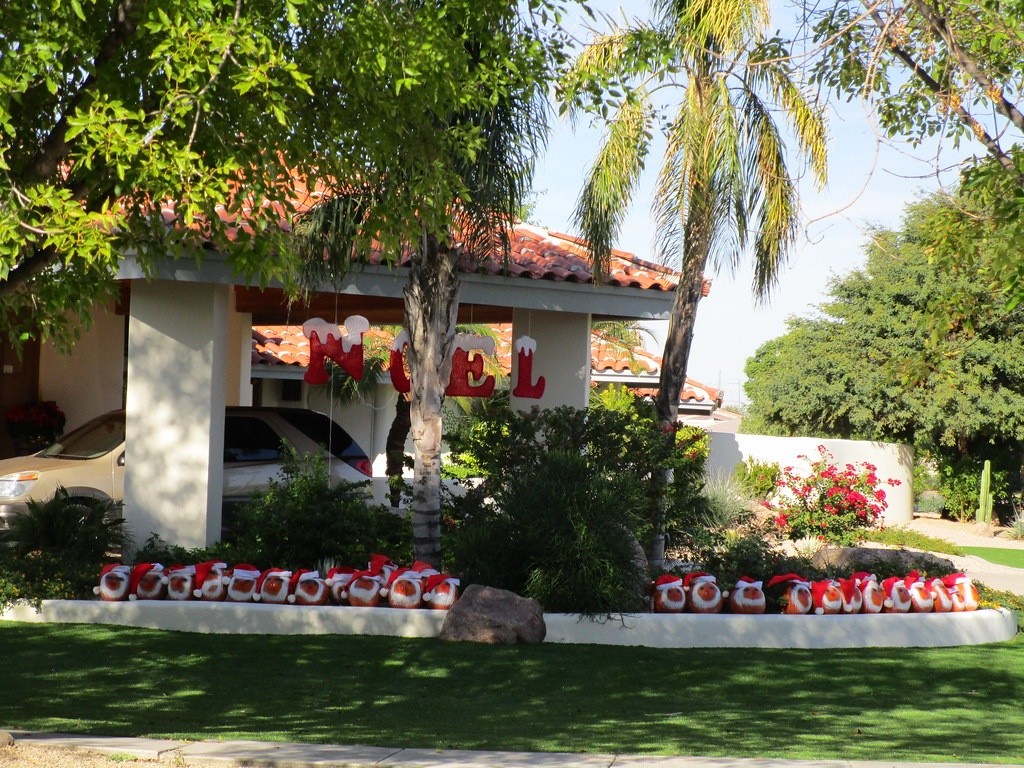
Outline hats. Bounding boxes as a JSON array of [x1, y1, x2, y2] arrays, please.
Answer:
[[722, 576, 763, 598], [836, 577, 857, 611], [222, 564, 259, 586], [341, 571, 378, 599], [649, 576, 682, 597], [367, 555, 397, 576], [193, 561, 227, 598], [325, 566, 358, 587], [162, 566, 194, 585], [253, 568, 292, 602], [93, 563, 128, 596], [768, 573, 808, 588], [128, 563, 164, 602], [809, 581, 833, 615], [287, 568, 319, 603], [422, 574, 460, 602], [411, 561, 440, 578], [851, 571, 877, 593], [683, 572, 716, 592], [381, 567, 421, 598], [882, 569, 977, 608]]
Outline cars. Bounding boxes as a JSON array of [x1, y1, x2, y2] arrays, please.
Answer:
[[0, 408, 373, 558]]
